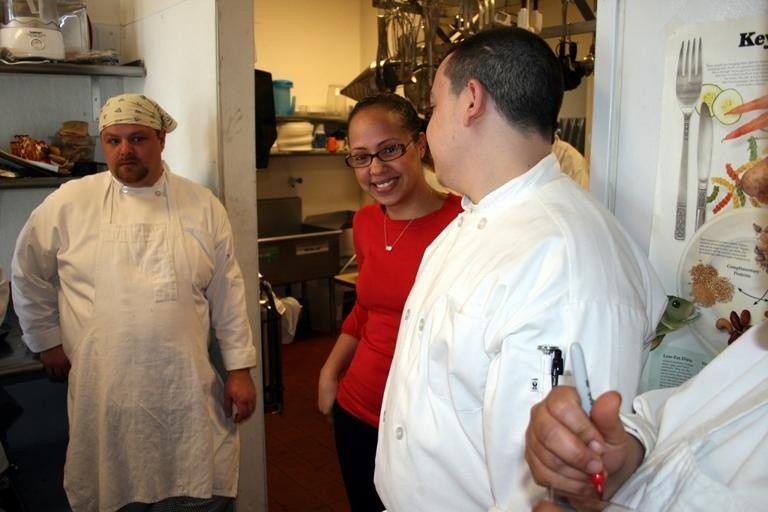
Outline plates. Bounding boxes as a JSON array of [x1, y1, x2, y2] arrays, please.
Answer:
[[678, 207, 768, 355]]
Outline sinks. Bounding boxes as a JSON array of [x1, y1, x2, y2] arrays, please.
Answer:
[[257, 225, 343, 243]]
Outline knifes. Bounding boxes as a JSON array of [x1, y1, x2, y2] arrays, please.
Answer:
[[696, 103, 712, 230]]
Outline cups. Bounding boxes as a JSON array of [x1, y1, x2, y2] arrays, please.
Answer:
[[314, 133, 340, 152], [272, 80, 295, 118]]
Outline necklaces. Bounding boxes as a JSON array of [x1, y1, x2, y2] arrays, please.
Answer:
[[382, 188, 435, 252]]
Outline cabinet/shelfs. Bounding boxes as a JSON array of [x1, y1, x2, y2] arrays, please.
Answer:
[[0, 57, 146, 190], [268, 111, 352, 158]]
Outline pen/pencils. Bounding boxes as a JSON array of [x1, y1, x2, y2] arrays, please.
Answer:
[[551, 349, 565, 390], [570, 343, 605, 500]]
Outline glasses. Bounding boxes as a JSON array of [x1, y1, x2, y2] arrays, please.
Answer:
[[344, 138, 411, 168]]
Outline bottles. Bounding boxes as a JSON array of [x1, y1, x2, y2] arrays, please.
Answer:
[[327, 83, 347, 112]]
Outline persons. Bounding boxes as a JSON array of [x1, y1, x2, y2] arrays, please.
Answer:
[[551, 124, 591, 190], [374, 23, 669, 512], [524, 316, 768, 512], [315, 90, 462, 512], [12, 94, 258, 512], [418, 118, 463, 200]]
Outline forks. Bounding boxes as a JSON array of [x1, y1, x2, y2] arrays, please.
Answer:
[[674, 37, 702, 241]]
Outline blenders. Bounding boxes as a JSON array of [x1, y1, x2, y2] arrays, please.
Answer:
[[1, 0, 68, 61]]
